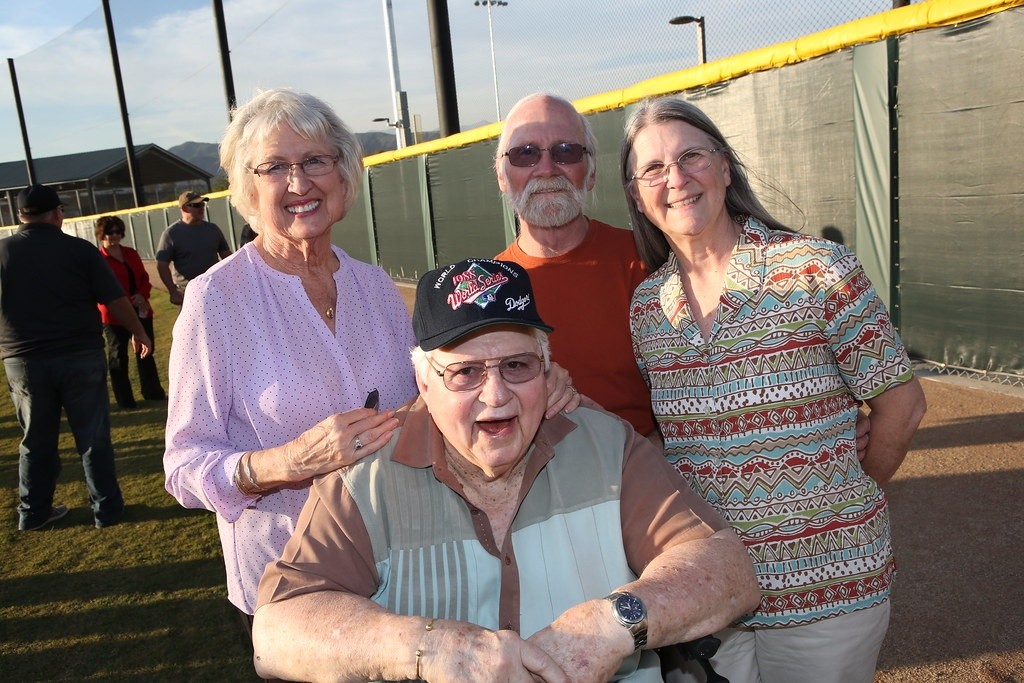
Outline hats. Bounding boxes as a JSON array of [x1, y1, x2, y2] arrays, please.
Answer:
[[179, 191, 210, 207], [18, 184, 62, 216], [414, 258, 552, 352]]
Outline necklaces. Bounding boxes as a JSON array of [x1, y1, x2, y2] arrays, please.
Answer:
[[301, 278, 334, 319]]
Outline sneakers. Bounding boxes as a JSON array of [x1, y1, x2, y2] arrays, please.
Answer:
[[94, 505, 124, 528], [18, 505, 68, 531]]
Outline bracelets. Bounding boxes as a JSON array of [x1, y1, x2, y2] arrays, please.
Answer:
[[233, 450, 269, 497], [416, 618, 436, 681]]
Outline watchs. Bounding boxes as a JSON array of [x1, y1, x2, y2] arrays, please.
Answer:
[[603, 592, 648, 652]]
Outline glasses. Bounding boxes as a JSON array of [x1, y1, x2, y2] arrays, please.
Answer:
[[56, 207, 64, 212], [247, 155, 340, 183], [186, 203, 205, 208], [105, 229, 122, 235], [502, 144, 590, 167], [631, 148, 720, 188], [425, 353, 545, 392]]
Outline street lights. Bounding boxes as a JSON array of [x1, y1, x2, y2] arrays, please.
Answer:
[[670, 12, 708, 63]]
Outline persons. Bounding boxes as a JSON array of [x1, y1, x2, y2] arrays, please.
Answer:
[[0, 183, 152, 532], [252, 257, 761, 683], [95, 216, 166, 408], [620, 98, 927, 683], [491, 92, 665, 449], [163, 88, 420, 645], [240, 223, 259, 249], [156, 191, 233, 313]]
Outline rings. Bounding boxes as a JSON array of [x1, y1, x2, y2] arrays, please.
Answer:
[[354, 436, 363, 449]]
